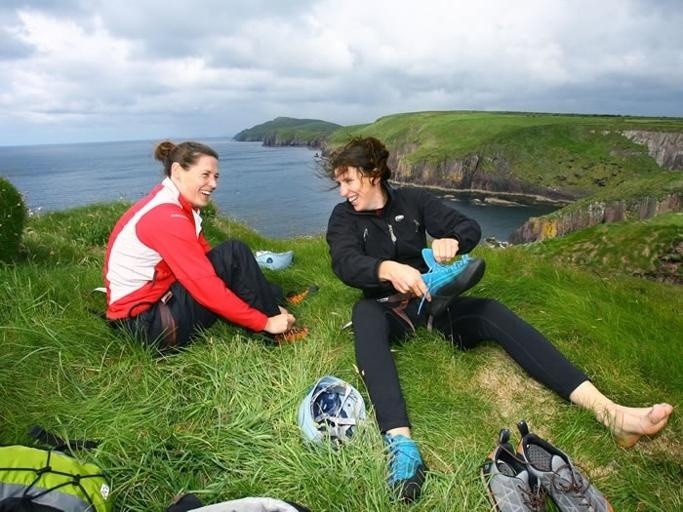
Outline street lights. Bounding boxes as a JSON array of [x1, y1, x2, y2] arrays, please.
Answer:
[[0, 446, 111, 512]]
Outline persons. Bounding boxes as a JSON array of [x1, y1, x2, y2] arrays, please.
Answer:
[[324, 133, 675, 507], [100, 139, 310, 355]]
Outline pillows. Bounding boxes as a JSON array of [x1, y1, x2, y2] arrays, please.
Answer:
[[298, 377, 366, 446]]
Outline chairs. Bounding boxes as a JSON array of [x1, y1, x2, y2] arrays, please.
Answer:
[[479, 430, 544, 512], [255, 248, 293, 270], [285, 289, 317, 305], [266, 326, 309, 343], [516, 421, 614, 511], [419, 247, 485, 315], [385, 436, 424, 500]]
